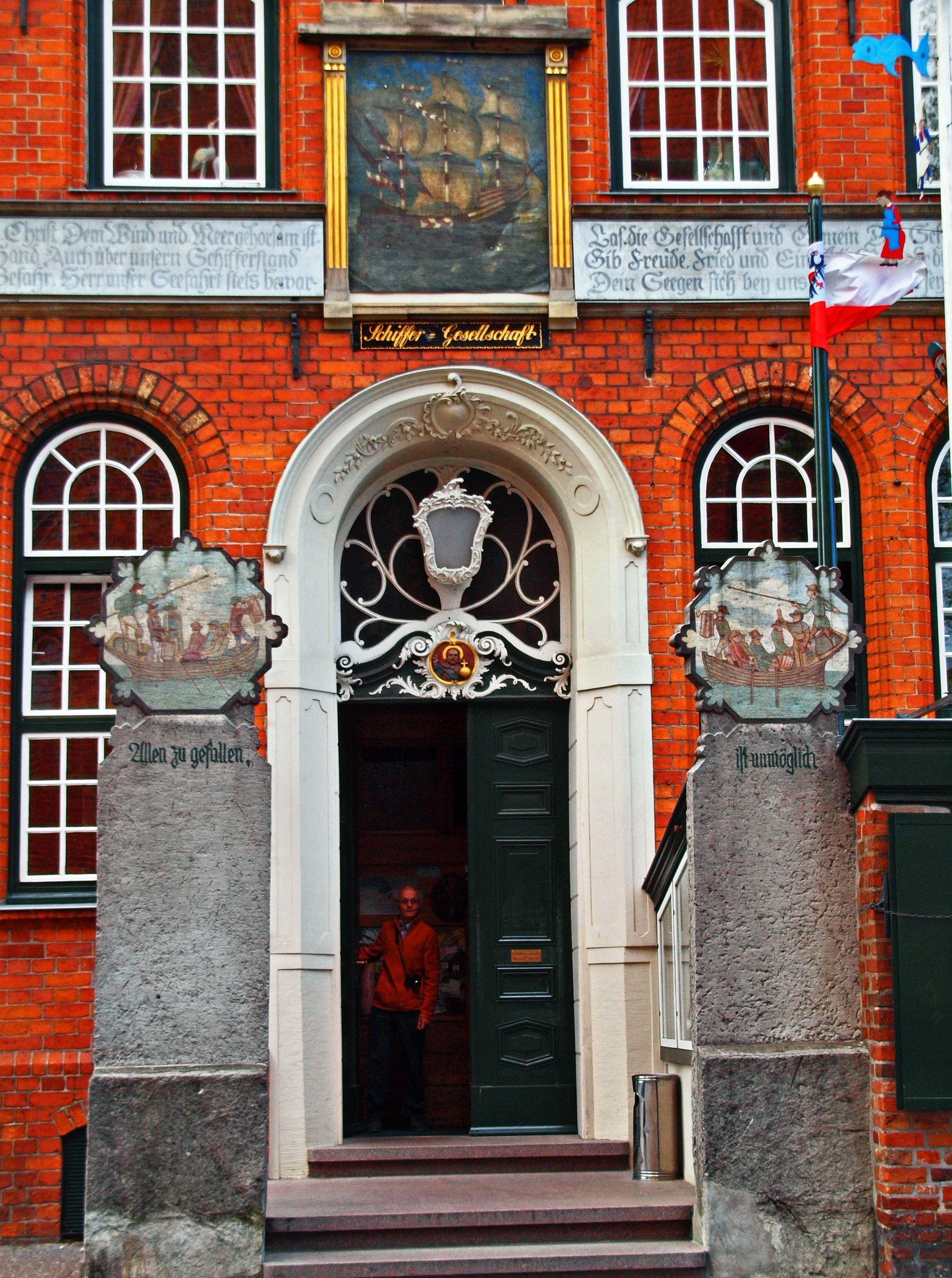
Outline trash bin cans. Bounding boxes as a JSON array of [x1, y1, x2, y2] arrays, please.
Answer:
[[632, 1073, 684, 1181]]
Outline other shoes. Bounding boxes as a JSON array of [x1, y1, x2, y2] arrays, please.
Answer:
[[408, 1117, 425, 1133], [364, 1118, 382, 1132]]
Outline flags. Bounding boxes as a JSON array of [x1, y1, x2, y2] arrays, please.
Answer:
[[807, 240, 929, 376]]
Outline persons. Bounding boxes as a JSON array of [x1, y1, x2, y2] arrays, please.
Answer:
[[356, 881, 441, 1133]]
